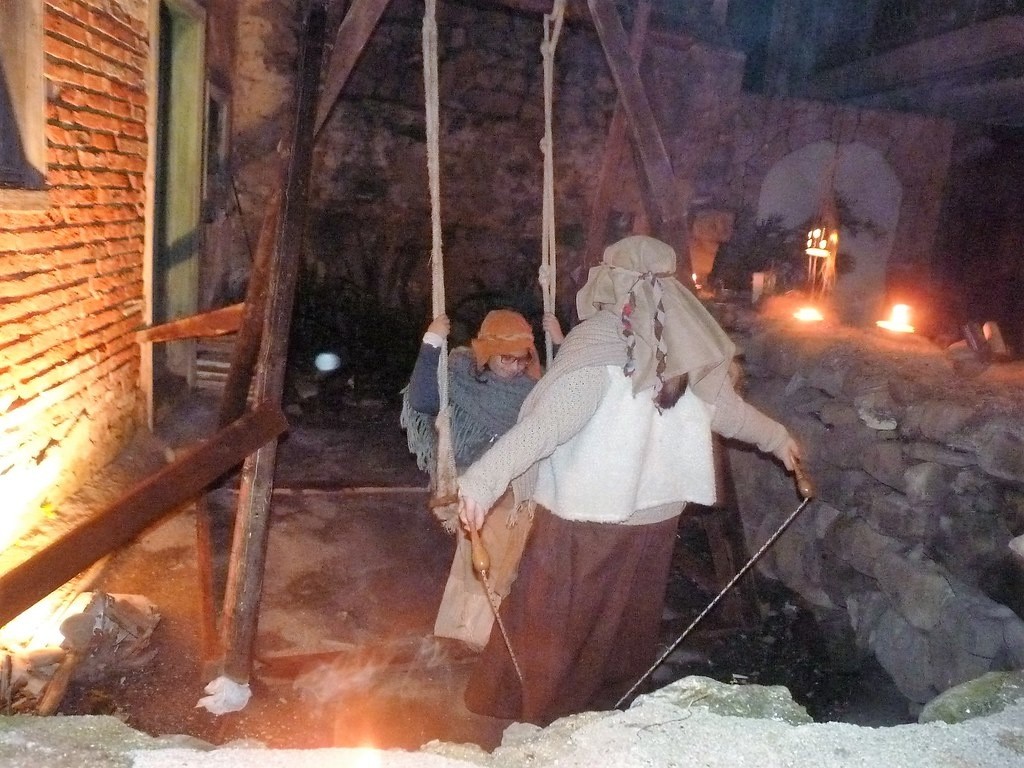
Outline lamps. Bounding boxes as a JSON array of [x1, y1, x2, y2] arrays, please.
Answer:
[[805, 225, 839, 257]]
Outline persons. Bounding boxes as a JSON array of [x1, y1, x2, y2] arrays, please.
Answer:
[[454, 235, 801, 728], [399, 309, 566, 655]]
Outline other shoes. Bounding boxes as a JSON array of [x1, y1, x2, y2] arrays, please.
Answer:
[[454, 647, 481, 664]]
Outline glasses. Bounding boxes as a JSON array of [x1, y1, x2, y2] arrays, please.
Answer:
[[497, 347, 533, 365]]
